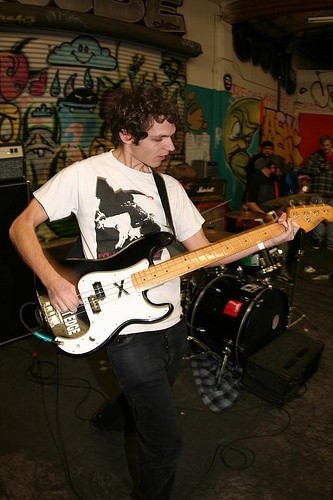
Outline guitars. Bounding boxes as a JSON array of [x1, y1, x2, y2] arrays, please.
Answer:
[[35, 198, 333, 359]]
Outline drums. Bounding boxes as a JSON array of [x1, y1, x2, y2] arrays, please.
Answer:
[[185, 272, 290, 373], [234, 246, 282, 275]]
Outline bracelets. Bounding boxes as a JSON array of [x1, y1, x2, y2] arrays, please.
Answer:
[[257, 242, 265, 250]]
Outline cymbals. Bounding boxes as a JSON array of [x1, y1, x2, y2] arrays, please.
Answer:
[[204, 229, 234, 242], [225, 211, 267, 219]]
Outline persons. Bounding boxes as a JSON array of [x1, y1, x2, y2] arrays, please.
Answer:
[[244, 141, 274, 180], [8, 87, 299, 500], [244, 156, 329, 286], [302, 135, 333, 198]]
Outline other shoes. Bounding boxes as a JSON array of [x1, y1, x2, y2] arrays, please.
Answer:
[[278, 271, 295, 286], [304, 266, 329, 281], [88, 411, 142, 438]]
